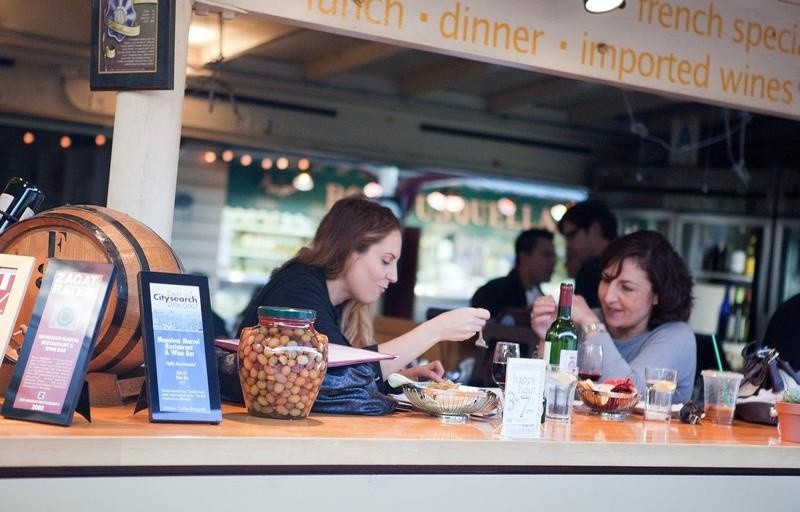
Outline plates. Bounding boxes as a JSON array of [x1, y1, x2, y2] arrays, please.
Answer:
[[634, 406, 679, 418]]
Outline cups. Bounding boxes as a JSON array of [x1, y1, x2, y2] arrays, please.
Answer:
[[701, 369, 745, 425], [544, 363, 579, 419], [644, 366, 679, 421]]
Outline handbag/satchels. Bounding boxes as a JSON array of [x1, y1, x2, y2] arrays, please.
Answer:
[[734, 340, 800, 426], [215, 343, 399, 417]]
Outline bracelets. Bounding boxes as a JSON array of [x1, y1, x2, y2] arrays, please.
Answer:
[[581, 321, 606, 339]]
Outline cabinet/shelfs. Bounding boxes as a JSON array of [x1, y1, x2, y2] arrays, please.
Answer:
[[611, 207, 773, 376]]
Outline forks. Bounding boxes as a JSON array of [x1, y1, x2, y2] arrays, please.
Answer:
[[474, 331, 490, 349]]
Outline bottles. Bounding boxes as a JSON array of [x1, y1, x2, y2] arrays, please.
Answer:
[[543, 281, 579, 369], [0, 176, 46, 236]]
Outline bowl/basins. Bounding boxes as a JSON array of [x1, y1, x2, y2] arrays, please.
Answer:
[[580, 383, 642, 416], [399, 383, 489, 421]]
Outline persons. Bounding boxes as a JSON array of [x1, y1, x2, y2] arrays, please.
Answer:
[[469, 198, 622, 317], [762, 292, 798, 378], [228, 197, 489, 402], [528, 230, 699, 410]]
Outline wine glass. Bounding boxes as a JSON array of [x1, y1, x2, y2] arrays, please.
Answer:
[[491, 339, 521, 408], [577, 341, 605, 414]]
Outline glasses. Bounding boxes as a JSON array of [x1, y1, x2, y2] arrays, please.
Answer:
[[679, 401, 703, 424], [565, 227, 583, 241]]
